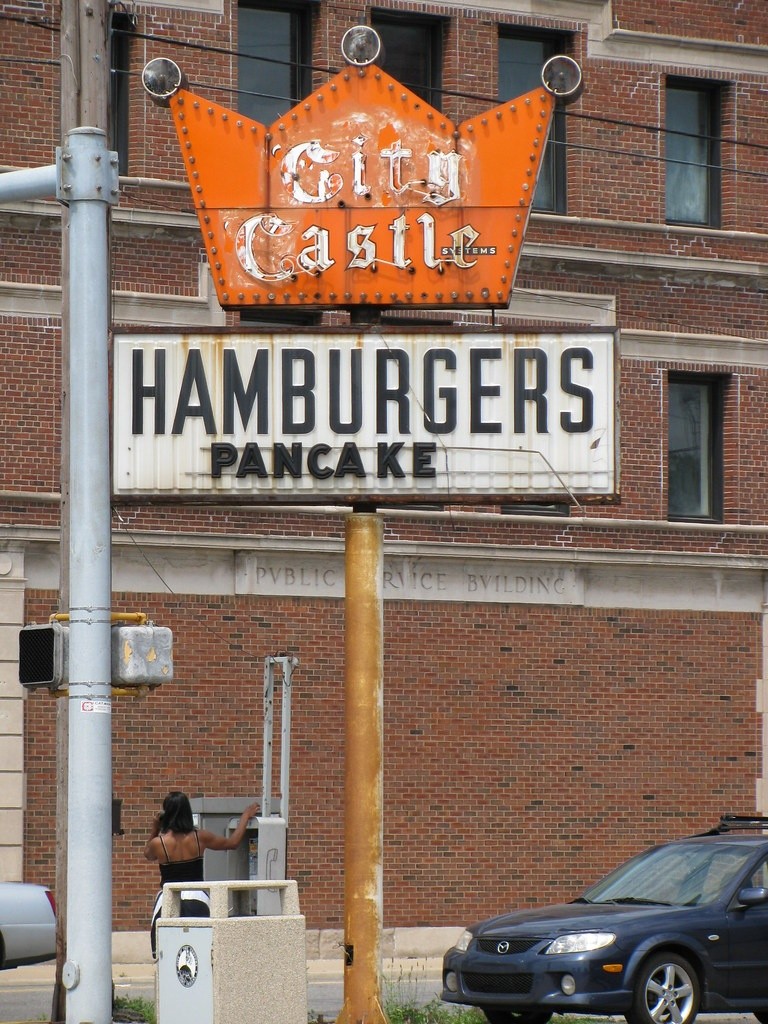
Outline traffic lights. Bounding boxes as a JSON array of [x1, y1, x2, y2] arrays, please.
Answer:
[[15, 622, 71, 689]]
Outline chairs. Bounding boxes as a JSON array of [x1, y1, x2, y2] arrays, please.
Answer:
[[722, 872, 753, 908]]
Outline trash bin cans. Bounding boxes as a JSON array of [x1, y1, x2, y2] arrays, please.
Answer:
[[154, 879, 308, 1024]]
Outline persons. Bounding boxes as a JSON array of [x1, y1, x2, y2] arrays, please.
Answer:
[[143, 790, 259, 962]]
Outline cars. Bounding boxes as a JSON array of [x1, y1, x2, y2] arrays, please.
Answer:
[[437, 809, 767, 1024]]
